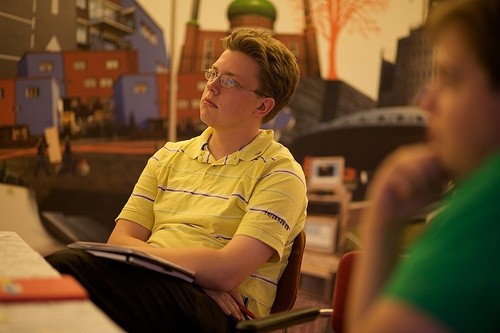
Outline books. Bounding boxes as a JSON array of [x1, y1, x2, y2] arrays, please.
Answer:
[[0, 229, 89, 302], [66, 240, 197, 284]]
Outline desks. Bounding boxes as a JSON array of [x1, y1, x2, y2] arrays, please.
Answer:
[[0, 230, 127, 333], [308, 192, 351, 253]]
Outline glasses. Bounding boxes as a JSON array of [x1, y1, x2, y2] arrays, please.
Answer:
[[203, 69, 259, 95]]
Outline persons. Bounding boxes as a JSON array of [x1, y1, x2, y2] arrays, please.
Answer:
[[44, 27, 307, 332], [344, 0, 499, 332]]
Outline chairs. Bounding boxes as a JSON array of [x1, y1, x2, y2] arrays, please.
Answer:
[[236, 230, 360, 333]]
